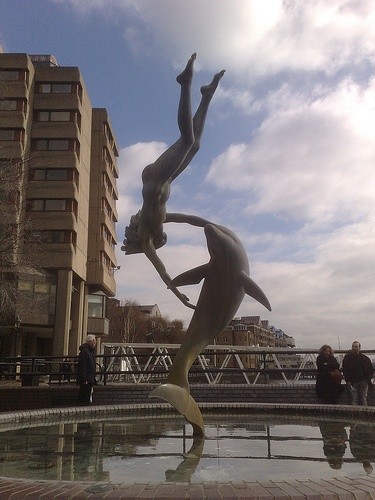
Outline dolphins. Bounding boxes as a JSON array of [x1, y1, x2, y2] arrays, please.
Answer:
[[162, 435, 204, 482], [148, 223, 272, 435]]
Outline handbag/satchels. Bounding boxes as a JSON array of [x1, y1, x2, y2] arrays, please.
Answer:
[[328, 368, 342, 385]]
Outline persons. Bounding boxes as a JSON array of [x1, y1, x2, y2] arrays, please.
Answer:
[[120, 52, 226, 309], [74, 423, 93, 477], [342, 341, 375, 406], [319, 422, 375, 474], [316, 345, 340, 404], [75, 334, 97, 406]]
